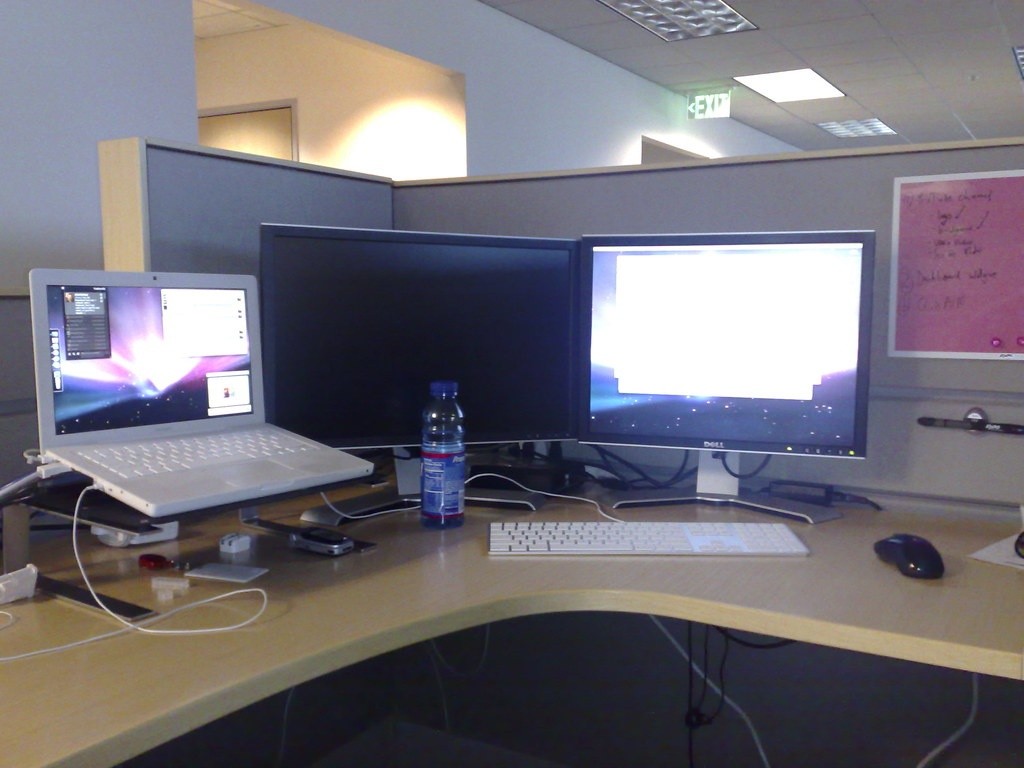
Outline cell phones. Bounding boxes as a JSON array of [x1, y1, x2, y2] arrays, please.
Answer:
[[289, 526, 355, 556]]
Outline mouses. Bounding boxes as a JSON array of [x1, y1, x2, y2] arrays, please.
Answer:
[[874, 532, 945, 580]]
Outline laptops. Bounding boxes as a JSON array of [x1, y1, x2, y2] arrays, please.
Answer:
[[27, 268, 374, 518]]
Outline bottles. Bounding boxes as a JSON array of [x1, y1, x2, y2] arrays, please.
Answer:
[[420, 381, 466, 528]]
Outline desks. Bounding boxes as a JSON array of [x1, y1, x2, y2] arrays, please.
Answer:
[[0, 492, 1024, 768]]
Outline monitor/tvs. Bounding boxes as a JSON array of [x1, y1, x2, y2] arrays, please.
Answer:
[[578, 229, 877, 524], [260, 223, 580, 529]]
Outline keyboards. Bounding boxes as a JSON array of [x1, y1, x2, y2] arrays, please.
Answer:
[[487, 522, 811, 556]]
